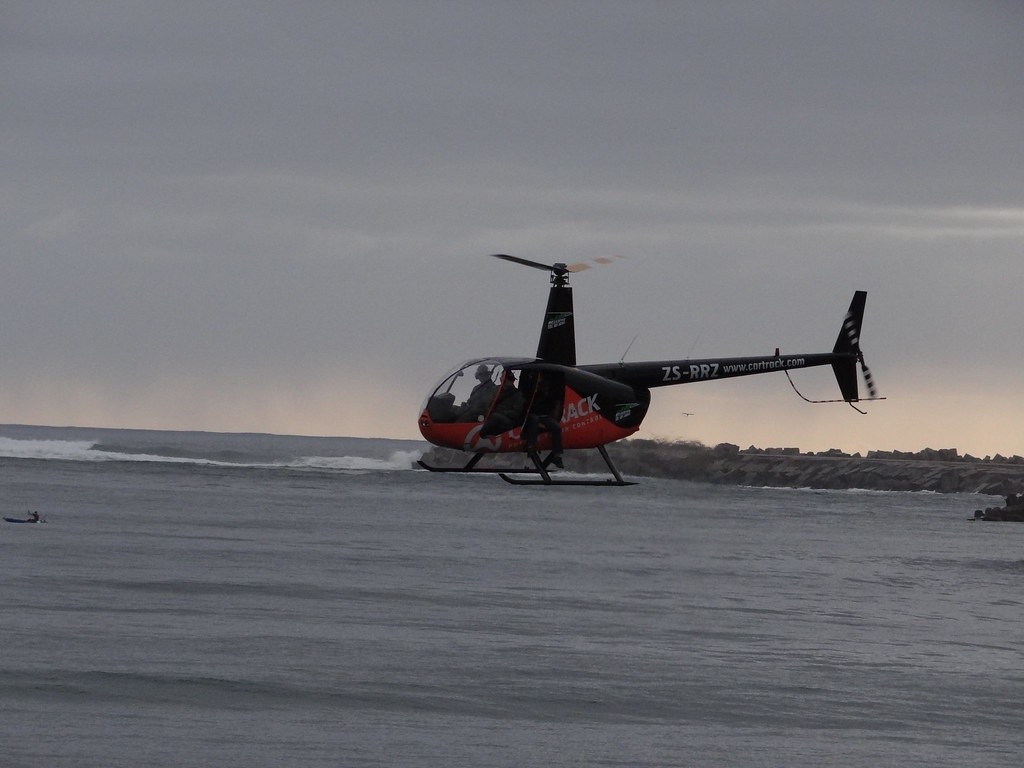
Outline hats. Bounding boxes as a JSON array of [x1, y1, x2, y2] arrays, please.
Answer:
[[501, 369, 517, 380], [474, 365, 489, 379]]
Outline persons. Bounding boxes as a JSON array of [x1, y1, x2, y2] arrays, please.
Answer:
[[457, 364, 523, 436]]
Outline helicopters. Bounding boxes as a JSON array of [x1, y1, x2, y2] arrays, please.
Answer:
[[416, 249, 888, 485]]
